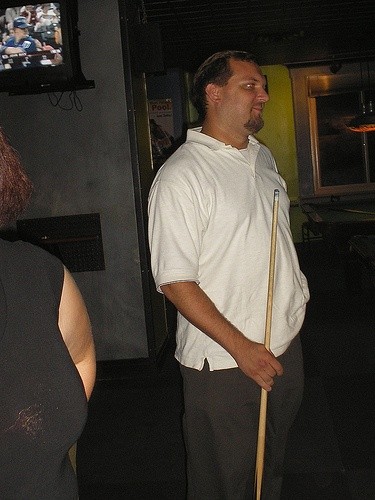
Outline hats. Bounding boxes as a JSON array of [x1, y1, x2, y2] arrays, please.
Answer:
[[13, 17, 32, 29]]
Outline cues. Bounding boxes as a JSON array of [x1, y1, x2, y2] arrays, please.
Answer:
[[252, 187, 282, 500]]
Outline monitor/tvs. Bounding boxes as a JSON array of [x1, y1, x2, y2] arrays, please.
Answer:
[[0, 0, 87, 92]]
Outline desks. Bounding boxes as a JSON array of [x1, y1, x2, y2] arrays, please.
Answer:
[[302, 198, 375, 284]]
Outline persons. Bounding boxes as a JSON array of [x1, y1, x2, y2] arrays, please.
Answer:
[[148, 50, 310, 500], [0, 139, 97, 500], [0, 1, 63, 70]]
